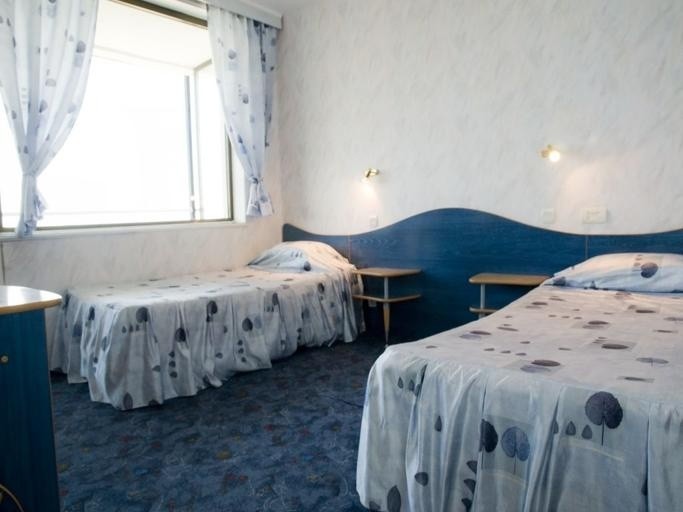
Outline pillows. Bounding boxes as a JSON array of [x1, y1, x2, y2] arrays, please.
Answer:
[[247, 241, 349, 275], [544, 252, 683, 293]]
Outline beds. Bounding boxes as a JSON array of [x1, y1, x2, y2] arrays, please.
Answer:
[[355, 280, 682, 510], [62, 264, 365, 409]]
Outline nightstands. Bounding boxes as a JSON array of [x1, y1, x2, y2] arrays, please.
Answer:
[[353, 267, 422, 349], [467, 272, 551, 319]]
